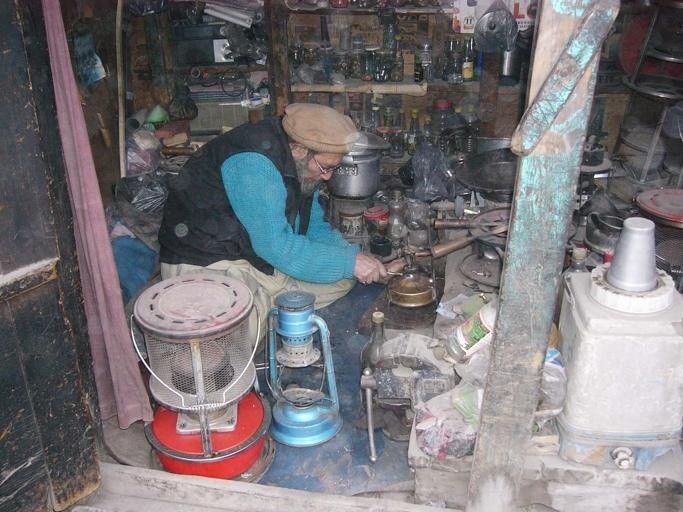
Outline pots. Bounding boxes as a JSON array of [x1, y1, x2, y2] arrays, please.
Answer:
[[327, 152, 381, 199]]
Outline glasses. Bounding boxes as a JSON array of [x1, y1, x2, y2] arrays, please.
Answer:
[[304, 148, 342, 175]]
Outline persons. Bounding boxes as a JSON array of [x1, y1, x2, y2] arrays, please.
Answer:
[[155, 100, 391, 352]]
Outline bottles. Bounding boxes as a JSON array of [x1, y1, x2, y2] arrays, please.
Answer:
[[554, 244, 591, 326], [360, 311, 388, 371], [317, 13, 474, 157], [387, 190, 408, 239], [446, 298, 498, 364]]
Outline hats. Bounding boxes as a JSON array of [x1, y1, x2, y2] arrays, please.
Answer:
[[281, 101, 361, 154]]
